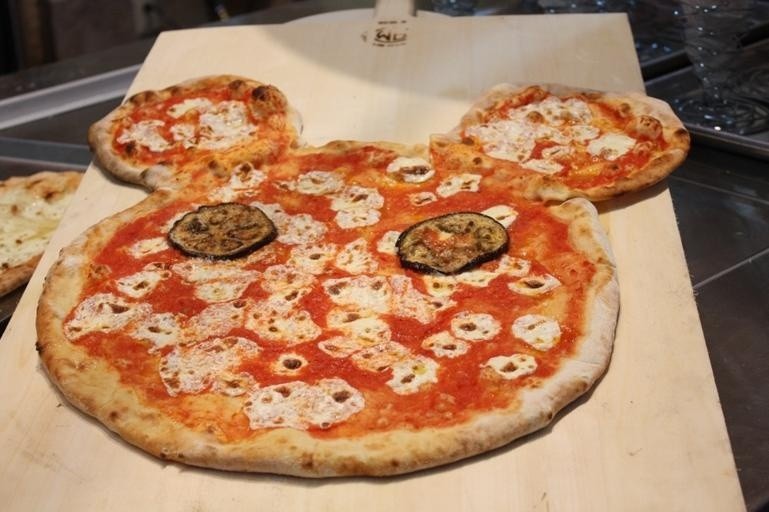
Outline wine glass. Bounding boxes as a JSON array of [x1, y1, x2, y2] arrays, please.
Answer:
[[671, 0, 768, 135]]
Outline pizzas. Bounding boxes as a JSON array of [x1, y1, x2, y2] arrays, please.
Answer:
[[1, 170, 83, 295]]
